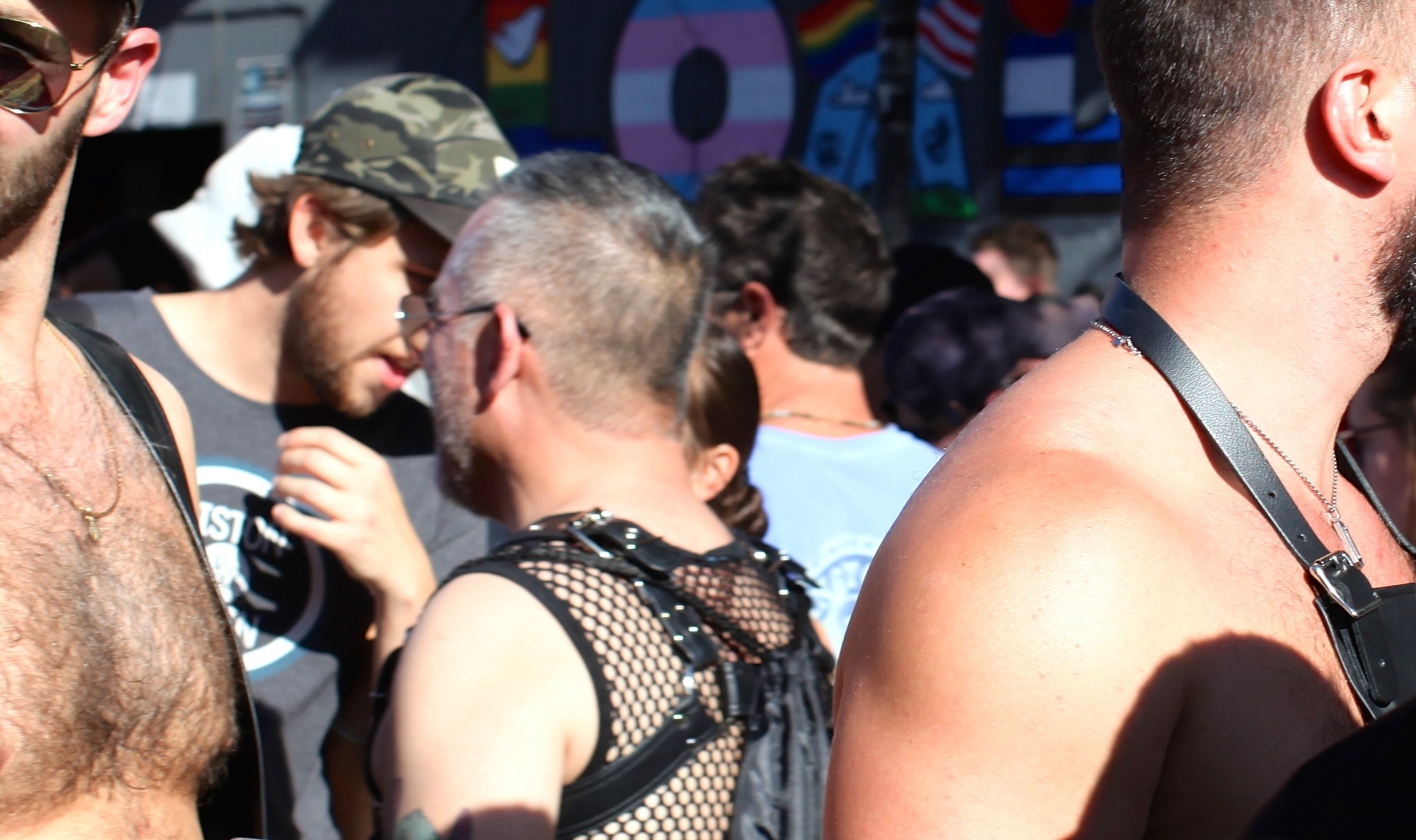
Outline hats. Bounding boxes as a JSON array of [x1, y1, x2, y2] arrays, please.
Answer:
[[290, 73, 520, 252]]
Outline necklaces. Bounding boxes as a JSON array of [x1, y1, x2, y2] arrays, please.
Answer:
[[1088, 319, 1369, 573], [2, 321, 122, 541], [760, 411, 881, 429]]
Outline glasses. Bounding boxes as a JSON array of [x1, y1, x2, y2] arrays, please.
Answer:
[[1, 18, 129, 114], [396, 291, 536, 370]]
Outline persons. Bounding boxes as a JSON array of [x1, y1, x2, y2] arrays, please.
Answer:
[[674, 158, 943, 665], [2, 0, 1416, 840]]
